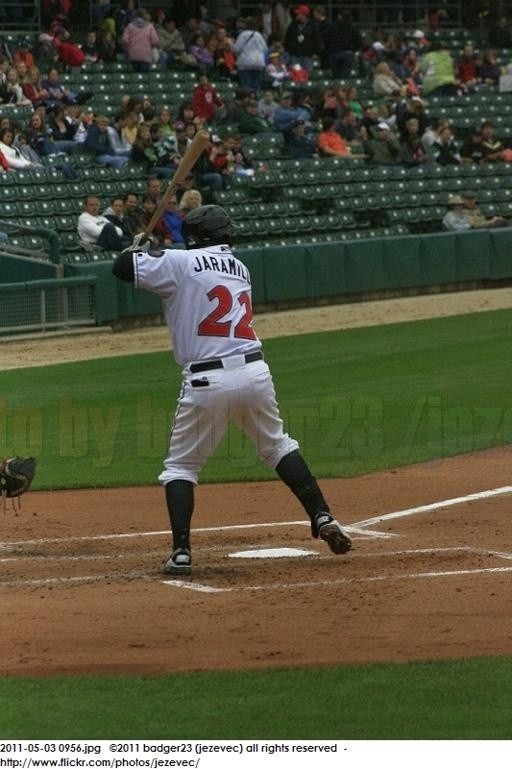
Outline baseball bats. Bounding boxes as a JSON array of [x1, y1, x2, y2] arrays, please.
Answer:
[[143, 129, 210, 236]]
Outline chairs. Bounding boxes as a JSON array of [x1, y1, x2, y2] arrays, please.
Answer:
[[1, 24, 512, 268]]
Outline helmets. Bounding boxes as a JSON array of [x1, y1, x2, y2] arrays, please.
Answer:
[[180, 204, 240, 250]]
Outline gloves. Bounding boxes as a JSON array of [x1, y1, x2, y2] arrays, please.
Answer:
[[121, 232, 154, 255]]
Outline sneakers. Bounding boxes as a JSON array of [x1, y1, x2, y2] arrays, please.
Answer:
[[161, 546, 193, 576], [314, 511, 354, 555]]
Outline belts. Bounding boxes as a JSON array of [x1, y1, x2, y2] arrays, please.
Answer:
[[189, 350, 263, 374]]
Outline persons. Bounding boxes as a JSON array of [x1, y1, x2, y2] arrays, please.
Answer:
[[111, 204, 352, 576]]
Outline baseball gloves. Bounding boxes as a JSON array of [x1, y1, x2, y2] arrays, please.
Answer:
[[0, 457, 37, 498]]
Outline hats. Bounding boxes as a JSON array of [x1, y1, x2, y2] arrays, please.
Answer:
[[446, 190, 479, 208], [100, 4, 425, 164]]
